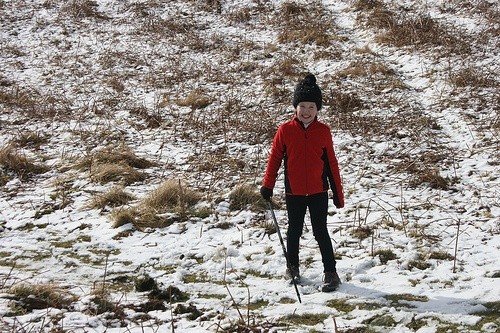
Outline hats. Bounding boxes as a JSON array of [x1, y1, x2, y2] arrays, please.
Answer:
[[293, 73, 322, 111]]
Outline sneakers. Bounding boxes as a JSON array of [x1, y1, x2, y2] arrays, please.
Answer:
[[285, 263, 301, 285], [321, 272, 342, 293]]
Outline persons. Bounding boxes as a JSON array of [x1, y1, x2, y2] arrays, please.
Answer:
[[260, 74, 344, 291]]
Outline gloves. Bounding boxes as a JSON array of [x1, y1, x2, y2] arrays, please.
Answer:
[[259, 186, 275, 202]]
